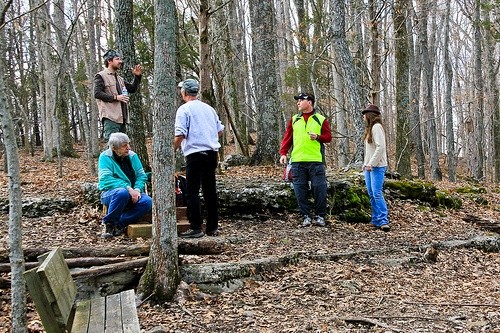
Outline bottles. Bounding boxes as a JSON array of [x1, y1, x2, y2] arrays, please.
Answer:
[[122, 87, 129, 102]]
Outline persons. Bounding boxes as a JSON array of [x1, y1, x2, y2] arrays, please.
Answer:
[[93, 48, 143, 148], [173, 78, 225, 237], [361, 104, 392, 230], [279, 92, 332, 227], [98, 131, 152, 238]]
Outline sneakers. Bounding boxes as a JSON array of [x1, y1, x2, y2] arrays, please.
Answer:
[[206, 229, 223, 237], [302, 214, 312, 227], [178, 228, 205, 238], [315, 215, 326, 227]]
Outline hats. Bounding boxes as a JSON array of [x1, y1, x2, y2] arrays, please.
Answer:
[[177, 78, 200, 93], [294, 93, 315, 104], [361, 104, 381, 115]]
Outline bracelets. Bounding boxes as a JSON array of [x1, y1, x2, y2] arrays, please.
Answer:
[[116, 93, 117, 100]]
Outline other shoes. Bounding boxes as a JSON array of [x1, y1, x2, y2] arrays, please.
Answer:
[[101, 222, 121, 241], [380, 225, 391, 231], [364, 223, 381, 229]]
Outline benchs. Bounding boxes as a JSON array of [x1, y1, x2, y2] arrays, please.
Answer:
[[22, 246, 140, 333]]
